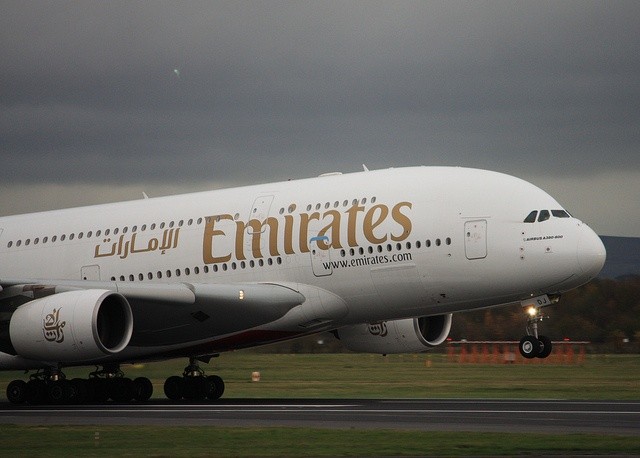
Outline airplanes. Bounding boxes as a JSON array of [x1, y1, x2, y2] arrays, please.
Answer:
[[0, 153, 606, 414]]
